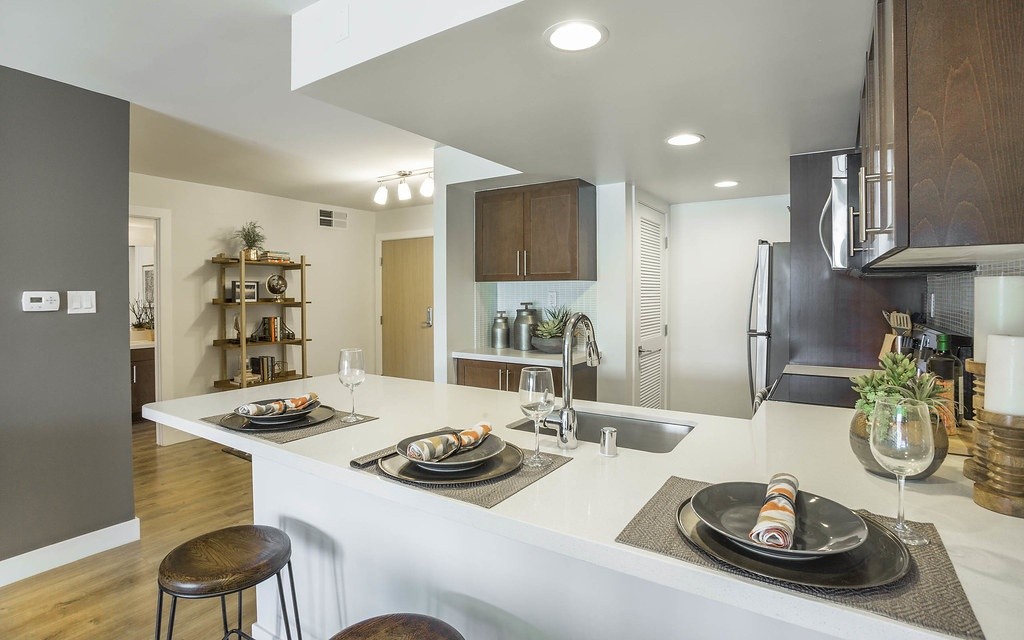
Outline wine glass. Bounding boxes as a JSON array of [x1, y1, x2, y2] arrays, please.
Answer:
[[868, 397, 935, 547], [519, 366, 555, 468], [337, 347, 366, 422]]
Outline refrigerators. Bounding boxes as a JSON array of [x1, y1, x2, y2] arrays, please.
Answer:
[[745, 239, 790, 410]]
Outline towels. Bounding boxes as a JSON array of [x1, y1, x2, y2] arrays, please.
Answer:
[[752, 387, 768, 418]]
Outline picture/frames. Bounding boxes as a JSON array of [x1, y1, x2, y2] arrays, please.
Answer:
[[232, 281, 260, 303], [142, 264, 156, 308]]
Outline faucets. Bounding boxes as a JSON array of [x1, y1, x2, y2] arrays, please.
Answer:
[[556, 311, 600, 450]]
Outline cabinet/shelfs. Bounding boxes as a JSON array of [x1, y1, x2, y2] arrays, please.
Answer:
[[131, 348, 154, 415], [208, 258, 311, 386], [475, 178, 597, 281], [456, 361, 563, 396], [849, 1, 1024, 273]]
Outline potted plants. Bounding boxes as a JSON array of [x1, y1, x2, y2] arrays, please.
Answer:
[[145, 300, 156, 341], [131, 294, 146, 330], [236, 222, 264, 260], [531, 308, 576, 353], [850, 358, 949, 482]]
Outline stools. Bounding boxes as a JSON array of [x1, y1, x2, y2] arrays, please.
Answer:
[[155, 526, 303, 640], [329, 613, 466, 640]]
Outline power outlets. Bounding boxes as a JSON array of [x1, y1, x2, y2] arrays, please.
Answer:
[[548, 291, 556, 306]]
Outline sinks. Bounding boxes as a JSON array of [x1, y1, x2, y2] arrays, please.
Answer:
[[505, 405, 697, 453]]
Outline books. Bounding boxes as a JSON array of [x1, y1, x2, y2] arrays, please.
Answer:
[[262, 316, 283, 342], [260, 251, 290, 263], [230, 355, 275, 386]]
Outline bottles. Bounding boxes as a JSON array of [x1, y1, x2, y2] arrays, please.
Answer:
[[925, 333, 965, 428]]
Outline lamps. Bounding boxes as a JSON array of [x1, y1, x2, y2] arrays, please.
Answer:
[[397, 176, 411, 201], [421, 172, 434, 197], [374, 181, 388, 205]]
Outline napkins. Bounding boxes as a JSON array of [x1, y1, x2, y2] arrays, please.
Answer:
[[751, 475, 802, 546], [408, 423, 493, 463], [239, 391, 317, 419]]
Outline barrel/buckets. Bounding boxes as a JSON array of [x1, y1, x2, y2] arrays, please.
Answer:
[[894, 335, 914, 357]]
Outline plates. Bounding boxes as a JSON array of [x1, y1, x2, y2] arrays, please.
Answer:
[[690, 481, 868, 561], [396, 428, 507, 473], [234, 396, 322, 425]]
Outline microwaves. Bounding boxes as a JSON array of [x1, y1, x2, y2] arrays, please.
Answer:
[[817, 152, 863, 276]]
